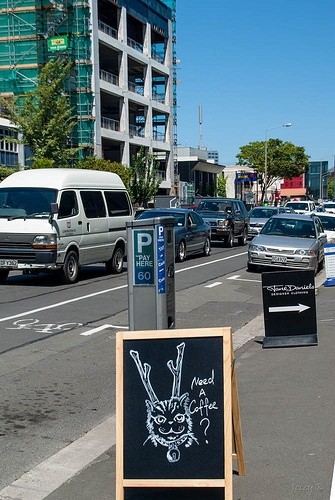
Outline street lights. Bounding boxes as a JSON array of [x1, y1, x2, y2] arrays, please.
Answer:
[[263, 122, 292, 204]]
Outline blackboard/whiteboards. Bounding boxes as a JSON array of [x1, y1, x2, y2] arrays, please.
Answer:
[[114, 327, 234, 500]]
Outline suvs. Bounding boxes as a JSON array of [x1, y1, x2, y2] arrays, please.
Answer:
[[196, 199, 250, 248]]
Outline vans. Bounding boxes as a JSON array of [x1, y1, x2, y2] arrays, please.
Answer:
[[0, 168, 135, 283]]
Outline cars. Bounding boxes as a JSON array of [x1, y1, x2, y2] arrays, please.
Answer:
[[134, 207, 212, 262], [246, 213, 328, 276], [249, 200, 335, 242]]
